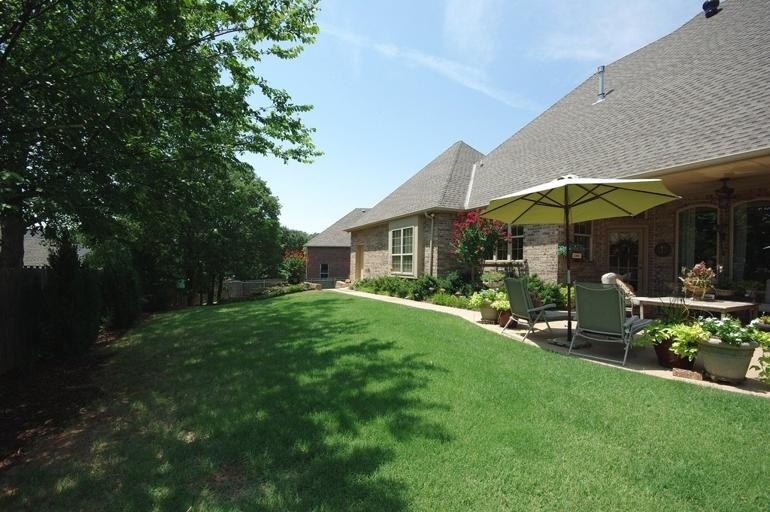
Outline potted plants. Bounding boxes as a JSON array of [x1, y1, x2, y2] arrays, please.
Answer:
[[740, 279, 764, 298], [716, 281, 736, 296], [467, 289, 504, 322], [491, 299, 519, 328], [696, 312, 770, 385], [633, 292, 699, 366], [557, 241, 587, 260]]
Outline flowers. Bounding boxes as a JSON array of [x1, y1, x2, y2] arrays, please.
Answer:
[[677, 262, 725, 290]]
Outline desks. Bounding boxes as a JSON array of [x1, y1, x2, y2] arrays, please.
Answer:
[[631, 296, 760, 326]]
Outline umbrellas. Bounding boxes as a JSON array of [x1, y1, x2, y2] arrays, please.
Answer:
[[479, 174, 683, 342]]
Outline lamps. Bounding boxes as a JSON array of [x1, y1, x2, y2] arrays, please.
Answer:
[[714, 177, 735, 211]]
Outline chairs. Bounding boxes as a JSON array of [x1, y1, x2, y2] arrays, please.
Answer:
[[502, 275, 557, 343], [568, 279, 653, 367]]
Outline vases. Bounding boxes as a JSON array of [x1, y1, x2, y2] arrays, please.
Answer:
[[686, 285, 717, 300]]
